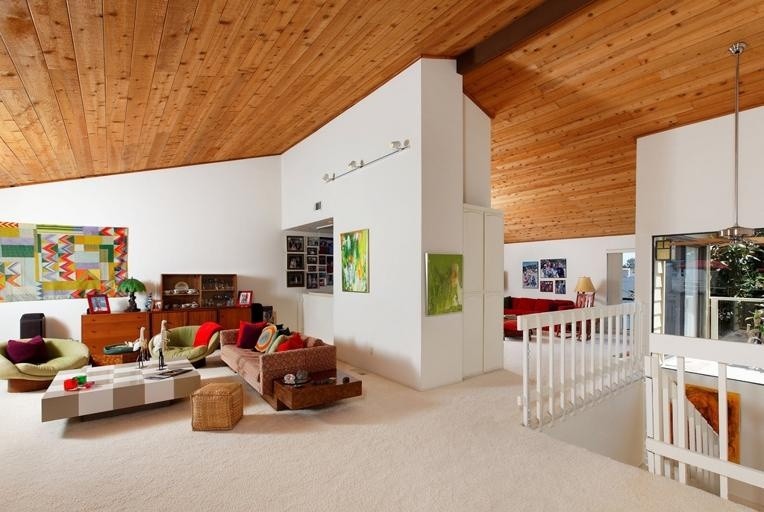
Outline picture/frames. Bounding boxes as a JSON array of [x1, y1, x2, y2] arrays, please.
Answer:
[[150, 300, 163, 312], [87, 293, 110, 314], [424, 250, 463, 316], [286, 234, 332, 290], [235, 290, 253, 306], [339, 227, 370, 294]]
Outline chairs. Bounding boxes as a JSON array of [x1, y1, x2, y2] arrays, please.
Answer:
[[555, 291, 596, 342]]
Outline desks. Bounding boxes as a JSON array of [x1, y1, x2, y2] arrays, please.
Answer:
[[273, 368, 363, 409]]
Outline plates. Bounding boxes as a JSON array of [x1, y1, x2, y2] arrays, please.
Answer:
[[280, 377, 310, 385], [174, 282, 188, 290]]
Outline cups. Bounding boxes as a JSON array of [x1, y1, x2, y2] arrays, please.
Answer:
[[165, 288, 195, 294], [164, 303, 198, 309], [64, 375, 86, 390]]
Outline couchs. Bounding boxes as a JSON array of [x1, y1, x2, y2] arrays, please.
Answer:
[[504, 296, 574, 333], [218, 321, 338, 395], [147, 325, 220, 362], [0, 335, 90, 393]]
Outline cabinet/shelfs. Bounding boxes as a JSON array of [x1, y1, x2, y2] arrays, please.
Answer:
[[151, 307, 252, 337], [161, 273, 237, 309], [80, 313, 149, 365]]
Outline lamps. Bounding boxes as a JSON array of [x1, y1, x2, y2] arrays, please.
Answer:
[[319, 139, 406, 183], [572, 276, 595, 308], [117, 276, 146, 312]]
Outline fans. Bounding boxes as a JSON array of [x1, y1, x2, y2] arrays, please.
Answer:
[[663, 42, 764, 247]]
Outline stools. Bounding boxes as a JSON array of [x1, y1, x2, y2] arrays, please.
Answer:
[[101, 351, 138, 365], [189, 382, 244, 430]]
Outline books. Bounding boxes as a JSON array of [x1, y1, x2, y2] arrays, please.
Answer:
[[145, 369, 192, 378]]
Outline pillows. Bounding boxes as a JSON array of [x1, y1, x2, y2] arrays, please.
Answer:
[[6, 336, 50, 364], [236, 321, 302, 356]]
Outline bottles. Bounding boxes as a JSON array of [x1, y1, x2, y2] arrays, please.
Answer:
[[144, 291, 153, 311], [207, 277, 229, 290]]
